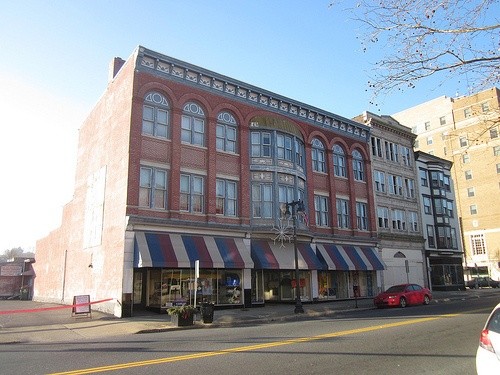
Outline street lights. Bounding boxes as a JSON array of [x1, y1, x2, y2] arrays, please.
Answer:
[[21, 259, 30, 286], [278, 198, 307, 314]]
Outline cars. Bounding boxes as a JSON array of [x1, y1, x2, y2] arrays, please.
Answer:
[[373, 284, 432, 309], [464, 277, 500, 289], [476, 303, 500, 375], [220, 285, 236, 297]]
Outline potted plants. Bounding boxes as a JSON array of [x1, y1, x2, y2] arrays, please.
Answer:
[[165, 302, 198, 326]]
